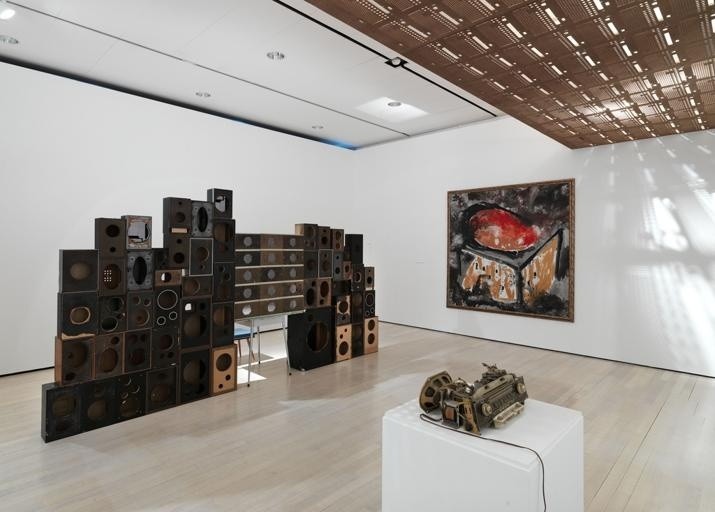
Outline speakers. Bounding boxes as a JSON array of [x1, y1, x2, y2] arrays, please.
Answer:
[[41, 186, 379, 445]]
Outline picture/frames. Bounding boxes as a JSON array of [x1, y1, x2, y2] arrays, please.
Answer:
[[446, 178, 575, 322]]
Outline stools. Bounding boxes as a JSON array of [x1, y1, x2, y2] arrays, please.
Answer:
[[234, 325, 261, 360]]
